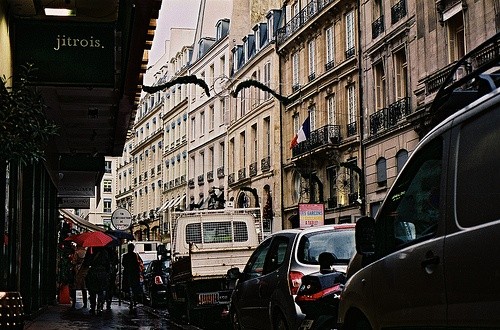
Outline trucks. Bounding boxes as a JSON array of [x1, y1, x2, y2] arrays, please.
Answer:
[[163, 208, 326, 328], [129, 241, 161, 262]]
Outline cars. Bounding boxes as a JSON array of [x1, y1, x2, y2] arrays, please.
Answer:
[[228, 223, 357, 329], [116, 258, 171, 308]]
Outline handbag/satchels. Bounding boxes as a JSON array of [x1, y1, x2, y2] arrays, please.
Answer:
[[137, 253, 144, 272], [57, 283, 71, 304]]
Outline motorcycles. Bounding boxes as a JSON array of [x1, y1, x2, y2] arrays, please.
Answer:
[[295, 251, 346, 330]]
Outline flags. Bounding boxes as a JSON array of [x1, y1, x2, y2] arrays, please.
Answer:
[[290, 116, 310, 150]]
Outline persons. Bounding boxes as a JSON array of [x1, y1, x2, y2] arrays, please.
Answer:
[[61, 243, 144, 313]]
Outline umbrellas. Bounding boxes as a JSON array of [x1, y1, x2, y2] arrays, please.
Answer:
[[64, 230, 136, 253]]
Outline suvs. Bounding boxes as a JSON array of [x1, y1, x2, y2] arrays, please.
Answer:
[[337, 34, 500, 327]]
